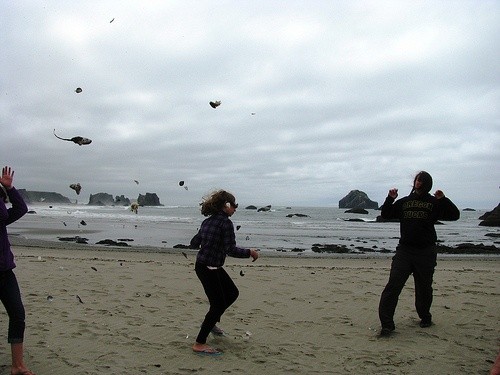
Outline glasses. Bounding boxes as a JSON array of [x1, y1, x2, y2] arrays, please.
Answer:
[[227, 201, 238, 208]]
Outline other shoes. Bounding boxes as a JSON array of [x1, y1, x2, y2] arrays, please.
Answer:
[[381, 325, 395, 335], [420, 314, 432, 327]]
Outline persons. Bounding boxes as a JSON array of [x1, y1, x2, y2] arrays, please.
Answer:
[[379, 171, 461, 335], [0, 164, 37, 375], [189, 189, 259, 355]]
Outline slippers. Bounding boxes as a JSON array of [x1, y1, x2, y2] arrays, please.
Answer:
[[11, 370, 36, 375], [212, 325, 223, 334], [193, 344, 219, 353]]
